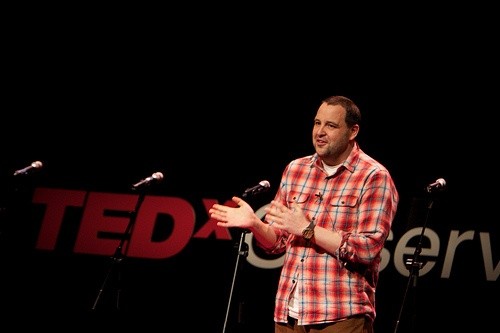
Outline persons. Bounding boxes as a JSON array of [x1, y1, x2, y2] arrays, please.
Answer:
[[209, 94, 400, 333]]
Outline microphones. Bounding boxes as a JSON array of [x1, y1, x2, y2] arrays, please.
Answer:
[[131, 171, 164, 193], [14, 161, 43, 177], [426, 178, 446, 193], [244, 180, 270, 199]]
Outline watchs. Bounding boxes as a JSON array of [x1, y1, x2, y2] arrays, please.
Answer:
[[303, 221, 316, 242]]
